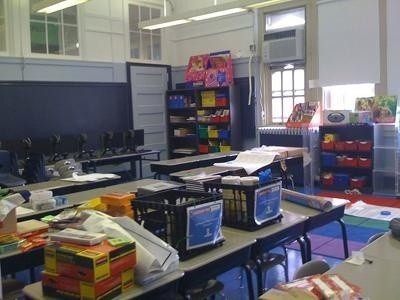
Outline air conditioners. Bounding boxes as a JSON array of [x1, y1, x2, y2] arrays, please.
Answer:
[[262, 23, 306, 70]]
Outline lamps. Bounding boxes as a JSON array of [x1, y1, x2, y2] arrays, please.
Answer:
[[138, 0, 287, 31], [35, 0, 88, 15]]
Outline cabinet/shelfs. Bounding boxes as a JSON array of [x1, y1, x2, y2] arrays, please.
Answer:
[[255, 127, 319, 186], [318, 124, 372, 194], [371, 124, 400, 198], [164, 84, 241, 159]]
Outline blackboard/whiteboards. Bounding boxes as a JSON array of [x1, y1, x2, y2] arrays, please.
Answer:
[[1, 80, 133, 163]]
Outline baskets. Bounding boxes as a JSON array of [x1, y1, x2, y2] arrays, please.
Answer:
[[131, 188, 223, 261], [203, 176, 283, 231]]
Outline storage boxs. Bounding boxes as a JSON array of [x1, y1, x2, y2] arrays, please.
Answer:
[[197, 91, 231, 154], [320, 140, 371, 151]]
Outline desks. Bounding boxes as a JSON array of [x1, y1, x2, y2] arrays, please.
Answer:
[[0, 148, 400, 300]]
